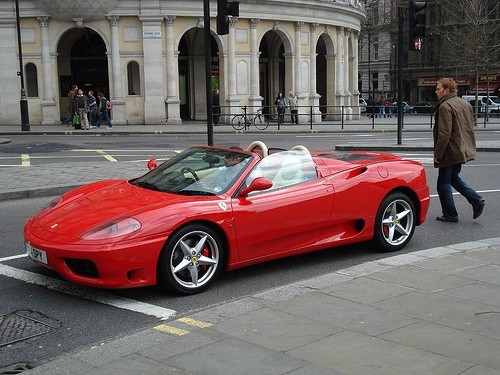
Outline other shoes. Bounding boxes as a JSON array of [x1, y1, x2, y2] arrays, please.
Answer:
[[64, 120, 69, 127], [436, 216, 458, 222], [90, 126, 95, 128], [473, 201, 485, 219], [106, 127, 112, 129], [82, 128, 89, 130]]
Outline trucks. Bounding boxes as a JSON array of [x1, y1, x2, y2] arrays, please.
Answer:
[[462, 95, 500, 116]]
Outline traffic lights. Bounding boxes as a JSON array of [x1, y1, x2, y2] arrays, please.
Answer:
[[409, 0, 426, 51], [216, 0, 239, 35]]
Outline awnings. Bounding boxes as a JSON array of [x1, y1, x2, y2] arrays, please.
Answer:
[[469, 82, 499, 93]]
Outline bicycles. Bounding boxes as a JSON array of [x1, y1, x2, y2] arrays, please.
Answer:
[[231, 107, 269, 130]]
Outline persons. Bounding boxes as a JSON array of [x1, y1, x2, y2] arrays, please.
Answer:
[[68, 85, 82, 129], [198, 146, 257, 197], [212, 89, 222, 126], [97, 93, 113, 129], [386, 96, 393, 118], [88, 91, 98, 129], [434, 77, 484, 222], [378, 96, 386, 118], [272, 156, 308, 190], [287, 91, 299, 125], [76, 90, 91, 130], [274, 92, 288, 125]]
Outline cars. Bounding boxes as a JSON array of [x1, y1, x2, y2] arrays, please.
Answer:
[[401, 101, 415, 113], [414, 101, 438, 113], [359, 98, 368, 111]]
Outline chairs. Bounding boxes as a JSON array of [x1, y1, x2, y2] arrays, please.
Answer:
[[266, 144, 317, 191], [247, 140, 270, 195]]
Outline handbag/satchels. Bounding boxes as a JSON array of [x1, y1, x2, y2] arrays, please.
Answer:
[[73, 113, 80, 124]]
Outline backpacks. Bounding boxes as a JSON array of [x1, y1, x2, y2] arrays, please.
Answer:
[[103, 98, 111, 109]]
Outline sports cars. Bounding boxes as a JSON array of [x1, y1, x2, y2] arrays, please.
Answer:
[[24, 140, 431, 296]]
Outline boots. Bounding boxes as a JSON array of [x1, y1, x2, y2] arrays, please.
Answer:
[[388, 114, 392, 118], [378, 114, 386, 118]]
